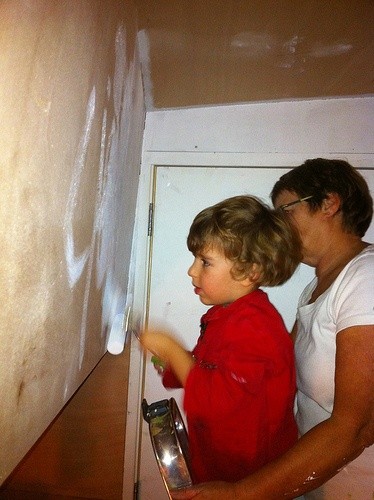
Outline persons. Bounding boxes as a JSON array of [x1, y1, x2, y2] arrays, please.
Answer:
[[165, 157, 374, 500], [134, 194, 306, 488]]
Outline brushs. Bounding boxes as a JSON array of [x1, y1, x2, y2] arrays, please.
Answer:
[[106, 313, 165, 368]]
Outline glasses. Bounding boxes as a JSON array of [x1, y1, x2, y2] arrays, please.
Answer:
[[277, 195, 315, 215]]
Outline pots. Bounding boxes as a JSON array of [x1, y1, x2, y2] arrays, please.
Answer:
[[141, 397, 193, 500]]
